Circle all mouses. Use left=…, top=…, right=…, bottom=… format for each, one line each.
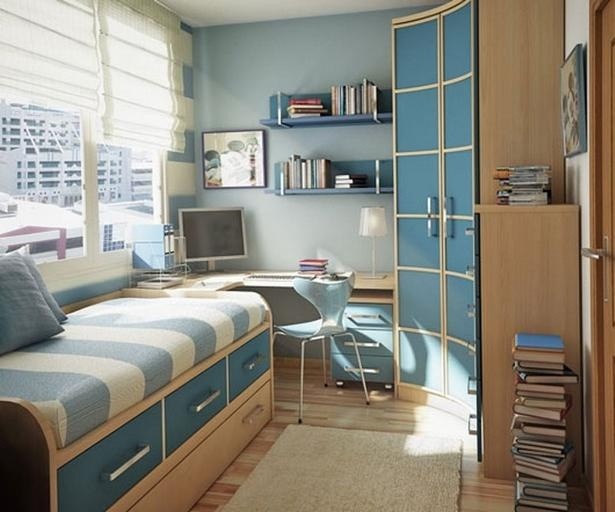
left=332, top=275, right=339, bottom=280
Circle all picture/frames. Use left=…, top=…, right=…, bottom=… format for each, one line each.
left=202, top=129, right=267, bottom=189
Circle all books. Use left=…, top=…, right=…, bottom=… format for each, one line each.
left=297, top=258, right=329, bottom=275
left=136, top=271, right=184, bottom=289
left=286, top=76, right=382, bottom=119
left=509, top=332, right=579, bottom=512
left=280, top=154, right=372, bottom=190
left=493, top=165, right=553, bottom=206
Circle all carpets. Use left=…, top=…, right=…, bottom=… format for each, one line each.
left=218, top=422, right=463, bottom=511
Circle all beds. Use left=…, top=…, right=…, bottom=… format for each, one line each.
left=0, top=287, right=273, bottom=512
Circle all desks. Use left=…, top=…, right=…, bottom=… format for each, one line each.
left=138, top=269, right=395, bottom=391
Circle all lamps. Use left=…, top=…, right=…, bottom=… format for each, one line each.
left=360, top=206, right=388, bottom=280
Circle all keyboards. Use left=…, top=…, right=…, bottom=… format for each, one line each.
left=243, top=274, right=316, bottom=282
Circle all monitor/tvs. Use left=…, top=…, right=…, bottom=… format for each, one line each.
left=178, top=206, right=249, bottom=274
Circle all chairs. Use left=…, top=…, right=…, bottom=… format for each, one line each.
left=270, top=273, right=370, bottom=424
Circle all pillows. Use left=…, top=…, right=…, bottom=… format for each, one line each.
left=0, top=242, right=69, bottom=355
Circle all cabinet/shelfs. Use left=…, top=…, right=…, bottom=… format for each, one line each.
left=391, top=2, right=564, bottom=415
left=466, top=201, right=582, bottom=480
